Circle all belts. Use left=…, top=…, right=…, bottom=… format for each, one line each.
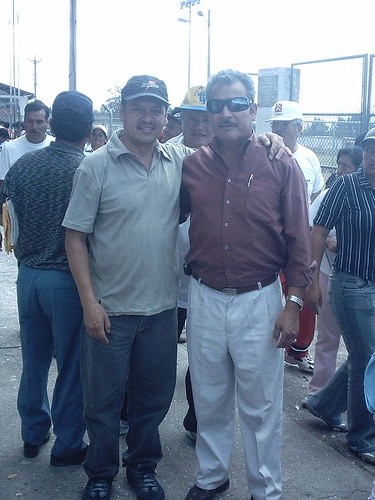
left=192, top=268, right=279, bottom=296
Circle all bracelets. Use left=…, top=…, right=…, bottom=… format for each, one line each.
left=286, top=293, right=305, bottom=308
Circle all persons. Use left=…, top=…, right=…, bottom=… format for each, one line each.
left=84, top=126, right=112, bottom=153
left=56, top=71, right=293, bottom=499
left=159, top=111, right=183, bottom=145
left=266, top=98, right=325, bottom=374
left=300, top=125, right=375, bottom=471
left=0, top=90, right=102, bottom=468
left=117, top=84, right=216, bottom=447
left=301, top=142, right=363, bottom=410
left=0, top=116, right=27, bottom=146
left=177, top=69, right=312, bottom=500
left=0, top=98, right=57, bottom=252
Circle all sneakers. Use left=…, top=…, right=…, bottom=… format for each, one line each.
left=126, top=466, right=164, bottom=500
left=83, top=477, right=112, bottom=500
left=284, top=350, right=314, bottom=372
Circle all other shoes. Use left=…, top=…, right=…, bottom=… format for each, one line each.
left=307, top=391, right=347, bottom=432
left=185, top=479, right=230, bottom=500
left=178, top=320, right=186, bottom=343
left=51, top=444, right=88, bottom=466
left=119, top=419, right=128, bottom=435
left=186, top=430, right=196, bottom=443
left=349, top=448, right=375, bottom=465
left=24, top=430, right=51, bottom=457
left=301, top=394, right=314, bottom=409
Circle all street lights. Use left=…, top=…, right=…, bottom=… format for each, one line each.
left=179, top=0, right=200, bottom=90
left=197, top=9, right=210, bottom=83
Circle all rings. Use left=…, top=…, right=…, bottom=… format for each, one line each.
left=291, top=338, right=298, bottom=344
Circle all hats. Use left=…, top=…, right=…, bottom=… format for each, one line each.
left=168, top=112, right=181, bottom=124
left=120, top=74, right=170, bottom=110
left=0, top=128, right=12, bottom=139
left=360, top=127, right=375, bottom=145
left=179, top=85, right=207, bottom=111
left=91, top=125, right=108, bottom=137
left=52, top=90, right=96, bottom=124
left=265, top=100, right=302, bottom=122
